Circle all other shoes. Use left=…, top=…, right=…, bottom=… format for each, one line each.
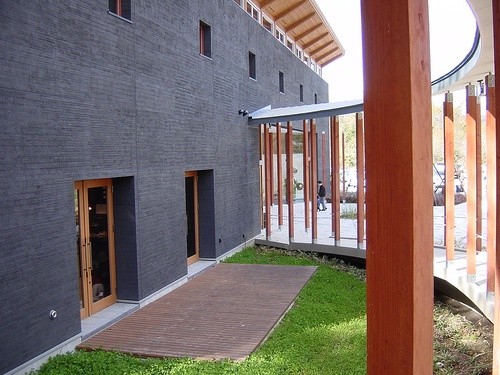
left=322, top=207, right=328, bottom=211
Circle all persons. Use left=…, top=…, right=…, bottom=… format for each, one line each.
left=317, top=194, right=321, bottom=211
left=317, top=181, right=328, bottom=211
